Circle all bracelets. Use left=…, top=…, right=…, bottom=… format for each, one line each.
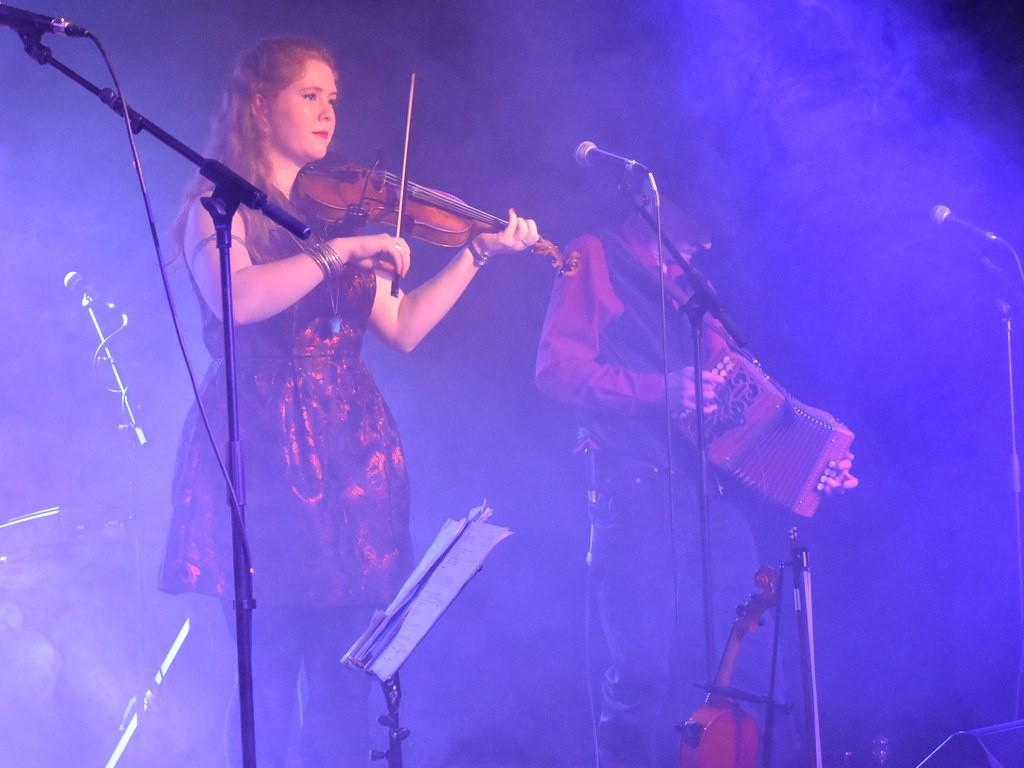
left=481, top=249, right=490, bottom=258
left=304, top=242, right=344, bottom=280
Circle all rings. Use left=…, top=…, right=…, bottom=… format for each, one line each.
left=521, top=240, right=528, bottom=247
left=394, top=243, right=402, bottom=250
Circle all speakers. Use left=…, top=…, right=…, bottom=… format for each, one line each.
left=916, top=719, right=1023, bottom=768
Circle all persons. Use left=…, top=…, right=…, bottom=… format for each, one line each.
left=158, top=35, right=538, bottom=767
left=534, top=144, right=858, bottom=767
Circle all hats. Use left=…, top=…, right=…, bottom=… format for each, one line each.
left=636, top=139, right=739, bottom=242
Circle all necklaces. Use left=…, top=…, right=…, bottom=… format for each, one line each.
left=279, top=225, right=342, bottom=336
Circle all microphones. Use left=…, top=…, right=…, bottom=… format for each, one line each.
left=64, top=271, right=118, bottom=312
left=573, top=140, right=650, bottom=176
left=0, top=3, right=89, bottom=37
left=930, top=205, right=1010, bottom=247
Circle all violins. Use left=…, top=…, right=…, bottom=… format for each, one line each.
left=674, top=565, right=780, bottom=768
left=295, top=152, right=583, bottom=279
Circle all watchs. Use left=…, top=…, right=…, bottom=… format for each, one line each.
left=467, top=241, right=488, bottom=268
